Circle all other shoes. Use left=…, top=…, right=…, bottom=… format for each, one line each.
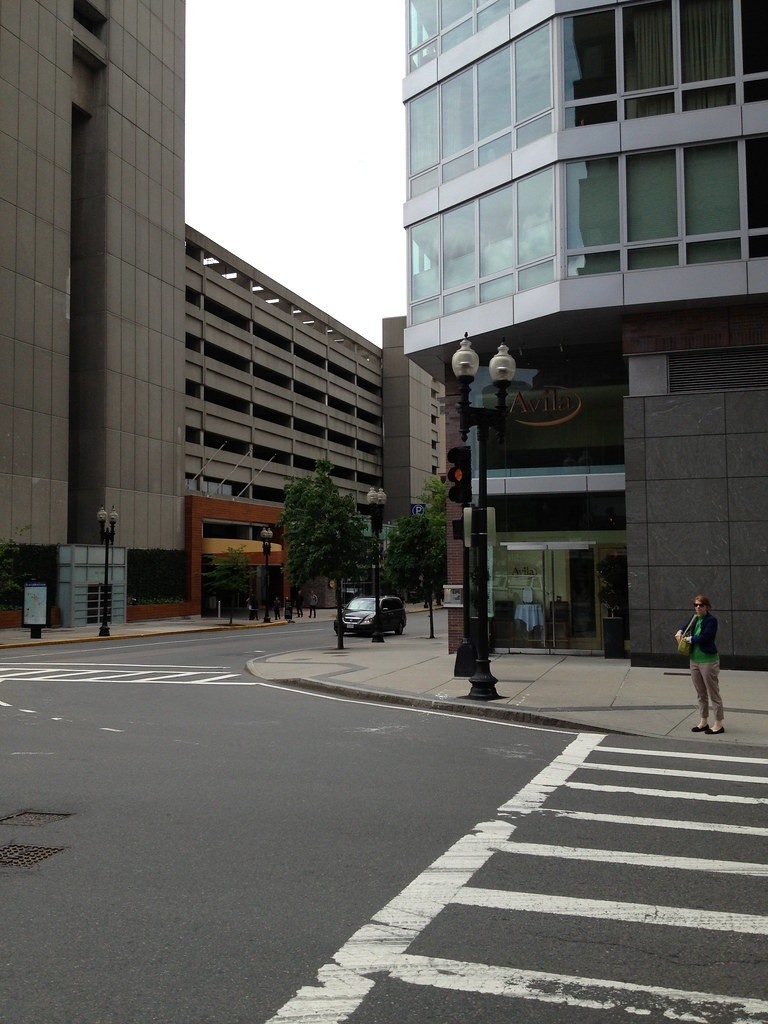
left=691, top=724, right=709, bottom=732
left=301, top=614, right=303, bottom=617
left=298, top=614, right=300, bottom=617
left=705, top=726, right=724, bottom=734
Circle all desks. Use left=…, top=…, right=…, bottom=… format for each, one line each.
left=514, top=604, right=544, bottom=648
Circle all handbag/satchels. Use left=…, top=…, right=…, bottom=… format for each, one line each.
left=678, top=614, right=696, bottom=656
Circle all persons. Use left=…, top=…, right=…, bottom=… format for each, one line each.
left=247, top=594, right=259, bottom=620
left=296, top=591, right=304, bottom=617
left=274, top=597, right=281, bottom=620
left=675, top=595, right=725, bottom=735
left=308, top=591, right=318, bottom=618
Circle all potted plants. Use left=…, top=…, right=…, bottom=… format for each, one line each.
left=596, top=555, right=628, bottom=659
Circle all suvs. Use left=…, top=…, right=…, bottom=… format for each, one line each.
left=333, top=595, right=407, bottom=636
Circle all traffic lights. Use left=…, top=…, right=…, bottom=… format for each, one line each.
left=446, top=446, right=473, bottom=504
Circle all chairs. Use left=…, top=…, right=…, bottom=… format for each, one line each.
left=491, top=599, right=515, bottom=648
left=546, top=600, right=570, bottom=646
left=527, top=604, right=548, bottom=648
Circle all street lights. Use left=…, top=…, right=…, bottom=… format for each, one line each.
left=260, top=528, right=273, bottom=623
left=366, top=487, right=386, bottom=643
left=450, top=329, right=518, bottom=699
left=99, top=505, right=119, bottom=636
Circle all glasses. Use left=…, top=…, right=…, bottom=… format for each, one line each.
left=693, top=603, right=706, bottom=607
